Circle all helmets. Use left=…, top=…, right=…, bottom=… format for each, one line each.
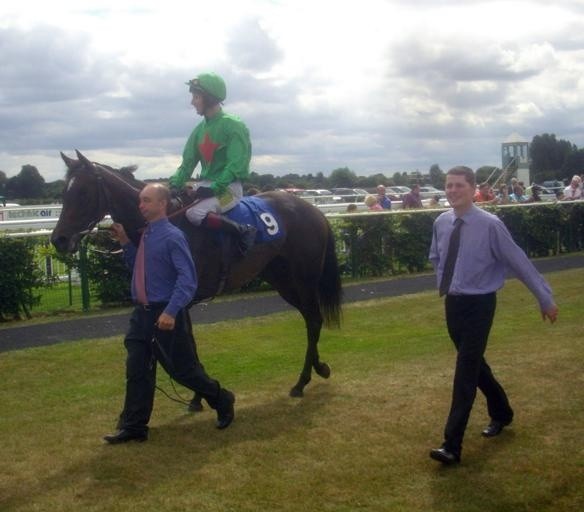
left=184, top=70, right=226, bottom=102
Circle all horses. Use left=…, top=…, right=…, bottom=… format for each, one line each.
left=49, top=147, right=344, bottom=414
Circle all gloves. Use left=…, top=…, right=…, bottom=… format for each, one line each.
left=197, top=186, right=213, bottom=199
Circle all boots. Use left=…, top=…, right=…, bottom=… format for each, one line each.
left=200, top=211, right=257, bottom=258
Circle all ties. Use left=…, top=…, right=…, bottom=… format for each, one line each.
left=439, top=218, right=464, bottom=297
left=135, top=227, right=149, bottom=305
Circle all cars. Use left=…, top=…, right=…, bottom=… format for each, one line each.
left=508, top=180, right=566, bottom=203
left=285, top=186, right=446, bottom=203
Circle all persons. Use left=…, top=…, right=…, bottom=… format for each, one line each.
left=162, top=73, right=257, bottom=259
left=102, top=182, right=235, bottom=444
left=344, top=183, right=442, bottom=212
left=474, top=175, right=584, bottom=206
left=428, top=166, right=558, bottom=464
left=242, top=182, right=278, bottom=197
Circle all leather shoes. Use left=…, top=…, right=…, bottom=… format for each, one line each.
left=482, top=417, right=513, bottom=437
left=104, top=427, right=149, bottom=444
left=216, top=388, right=235, bottom=430
left=430, top=445, right=462, bottom=466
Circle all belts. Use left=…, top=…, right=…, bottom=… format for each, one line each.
left=142, top=303, right=165, bottom=311
